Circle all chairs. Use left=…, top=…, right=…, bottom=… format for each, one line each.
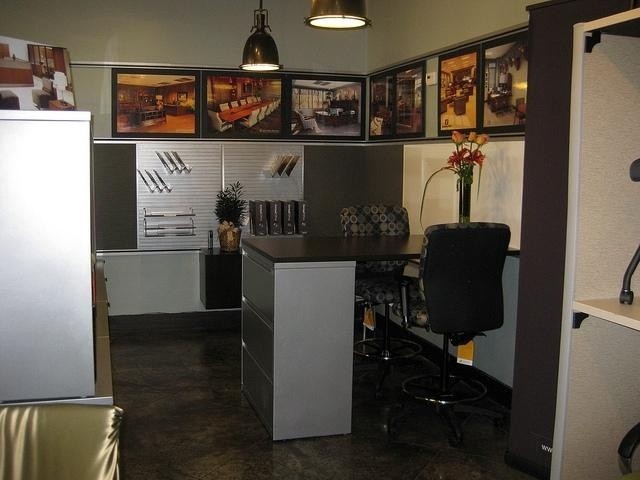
left=0, top=403, right=124, bottom=480
left=340, top=201, right=422, bottom=361
left=391, top=221, right=511, bottom=405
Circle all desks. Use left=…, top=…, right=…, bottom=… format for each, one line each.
left=32, top=89, right=74, bottom=110
left=0, top=90, right=20, bottom=110
left=241, top=234, right=522, bottom=441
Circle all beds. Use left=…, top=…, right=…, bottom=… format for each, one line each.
left=0, top=43, right=34, bottom=87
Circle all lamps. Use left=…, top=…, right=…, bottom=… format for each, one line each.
left=303, top=0, right=374, bottom=31
left=239, top=0, right=284, bottom=71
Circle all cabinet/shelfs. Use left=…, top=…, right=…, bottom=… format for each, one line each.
left=550, top=8, right=640, bottom=480
left=27, top=43, right=67, bottom=81
left=199, top=248, right=242, bottom=310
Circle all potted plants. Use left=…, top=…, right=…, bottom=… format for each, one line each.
left=213, top=179, right=249, bottom=253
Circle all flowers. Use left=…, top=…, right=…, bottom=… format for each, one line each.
left=417, top=131, right=490, bottom=227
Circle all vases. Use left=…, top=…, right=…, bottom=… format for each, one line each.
left=459, top=174, right=472, bottom=224
